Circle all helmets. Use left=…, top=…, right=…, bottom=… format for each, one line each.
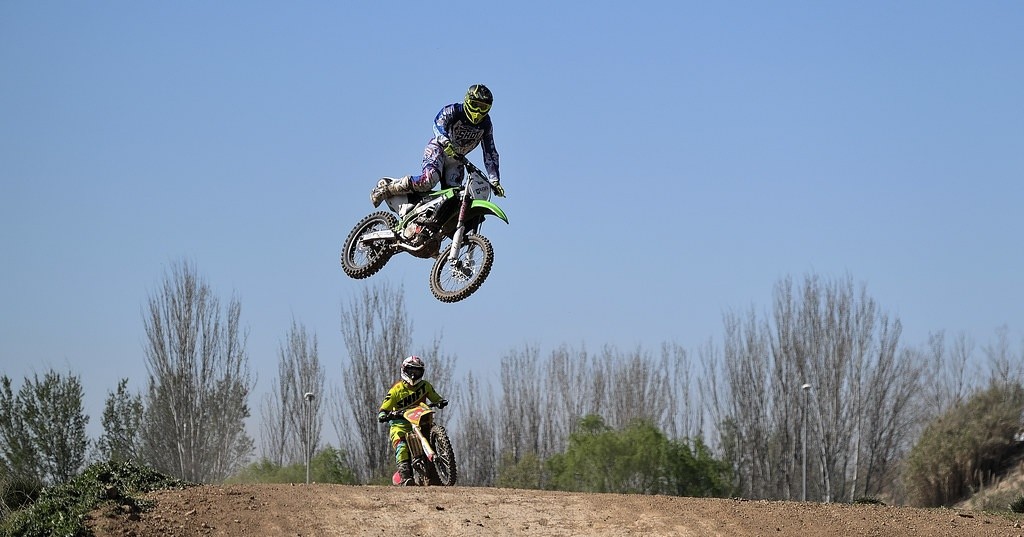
left=400, top=355, right=425, bottom=386
left=463, top=84, right=493, bottom=124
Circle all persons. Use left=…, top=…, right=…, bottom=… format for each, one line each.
left=379, top=356, right=448, bottom=486
left=371, top=85, right=504, bottom=207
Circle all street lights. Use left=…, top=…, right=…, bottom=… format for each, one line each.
left=801, top=383, right=814, bottom=502
left=303, top=391, right=316, bottom=484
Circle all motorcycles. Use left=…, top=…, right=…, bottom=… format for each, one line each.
left=340, top=146, right=509, bottom=304
left=379, top=401, right=458, bottom=487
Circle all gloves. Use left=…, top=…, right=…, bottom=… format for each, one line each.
left=437, top=400, right=448, bottom=409
left=378, top=411, right=387, bottom=423
left=444, top=143, right=455, bottom=158
left=492, top=181, right=504, bottom=197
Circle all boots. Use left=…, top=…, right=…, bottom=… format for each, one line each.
left=370, top=176, right=409, bottom=208
left=397, top=460, right=415, bottom=487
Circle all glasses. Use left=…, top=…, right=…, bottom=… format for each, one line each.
left=405, top=367, right=423, bottom=377
left=469, top=100, right=491, bottom=112
